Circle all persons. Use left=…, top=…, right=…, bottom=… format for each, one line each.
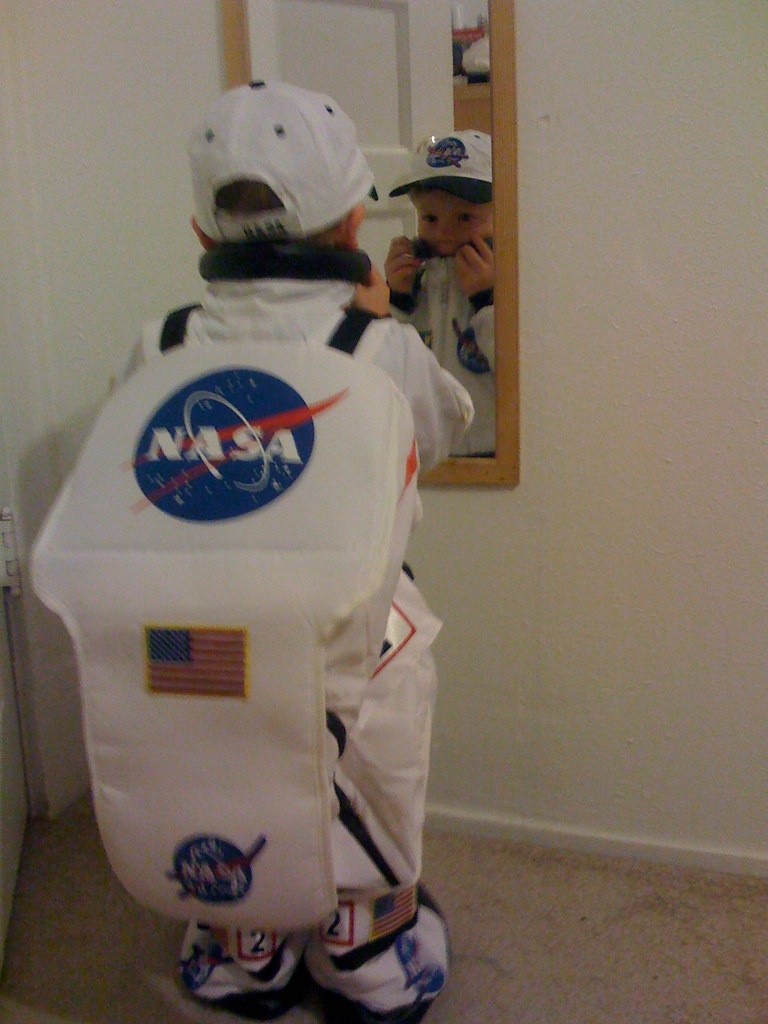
left=383, top=129, right=496, bottom=458
left=28, top=80, right=475, bottom=1024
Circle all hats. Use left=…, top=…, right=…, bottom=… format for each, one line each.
left=388, top=128, right=492, bottom=204
left=187, top=79, right=378, bottom=243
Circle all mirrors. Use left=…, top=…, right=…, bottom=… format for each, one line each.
left=221, top=0, right=521, bottom=493
